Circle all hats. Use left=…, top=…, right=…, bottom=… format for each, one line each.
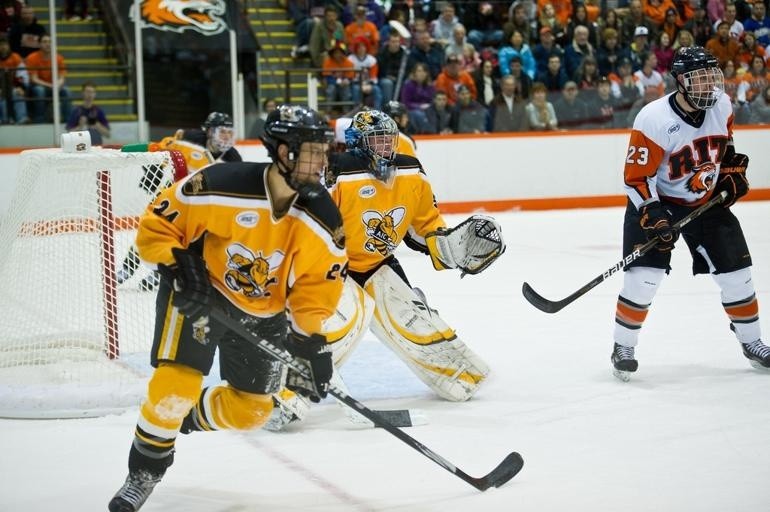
left=634, top=26, right=648, bottom=36
left=446, top=56, right=459, bottom=64
left=564, top=81, right=576, bottom=89
left=541, top=27, right=551, bottom=34
left=666, top=6, right=676, bottom=16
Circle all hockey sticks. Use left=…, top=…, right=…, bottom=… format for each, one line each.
left=330, top=366, right=431, bottom=428
left=390, top=20, right=411, bottom=101
left=158, top=262, right=524, bottom=491
left=522, top=189, right=729, bottom=314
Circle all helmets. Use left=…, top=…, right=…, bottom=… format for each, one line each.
left=671, top=46, right=723, bottom=111
left=260, top=104, right=336, bottom=196
left=345, top=109, right=399, bottom=190
left=204, top=112, right=235, bottom=152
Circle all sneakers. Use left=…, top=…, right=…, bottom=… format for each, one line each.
left=141, top=269, right=162, bottom=291
left=114, top=268, right=134, bottom=282
left=742, top=340, right=770, bottom=366
left=611, top=343, right=637, bottom=374
left=109, top=472, right=164, bottom=512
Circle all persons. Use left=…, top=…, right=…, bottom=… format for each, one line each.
left=0, top=0, right=68, bottom=125
left=261, top=109, right=506, bottom=431
left=260, top=99, right=276, bottom=121
left=298, top=1, right=769, bottom=134
left=65, top=82, right=112, bottom=146
left=115, top=111, right=244, bottom=290
left=327, top=101, right=437, bottom=256
left=611, top=46, right=770, bottom=381
left=108, top=106, right=350, bottom=512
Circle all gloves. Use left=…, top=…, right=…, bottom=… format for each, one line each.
left=426, top=216, right=508, bottom=274
left=720, top=154, right=750, bottom=208
left=160, top=245, right=215, bottom=321
left=641, top=201, right=679, bottom=252
left=282, top=331, right=332, bottom=404
